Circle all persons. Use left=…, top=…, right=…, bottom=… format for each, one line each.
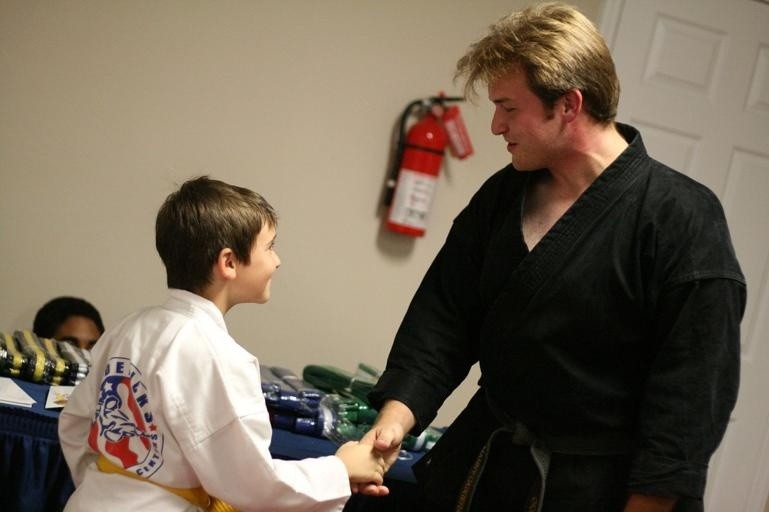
left=33, top=296, right=104, bottom=351
left=57, top=174, right=386, bottom=512
left=350, top=0, right=747, bottom=512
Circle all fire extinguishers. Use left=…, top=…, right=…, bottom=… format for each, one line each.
left=381, top=94, right=474, bottom=238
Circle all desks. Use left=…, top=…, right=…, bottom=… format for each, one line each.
left=0, top=377, right=449, bottom=511
